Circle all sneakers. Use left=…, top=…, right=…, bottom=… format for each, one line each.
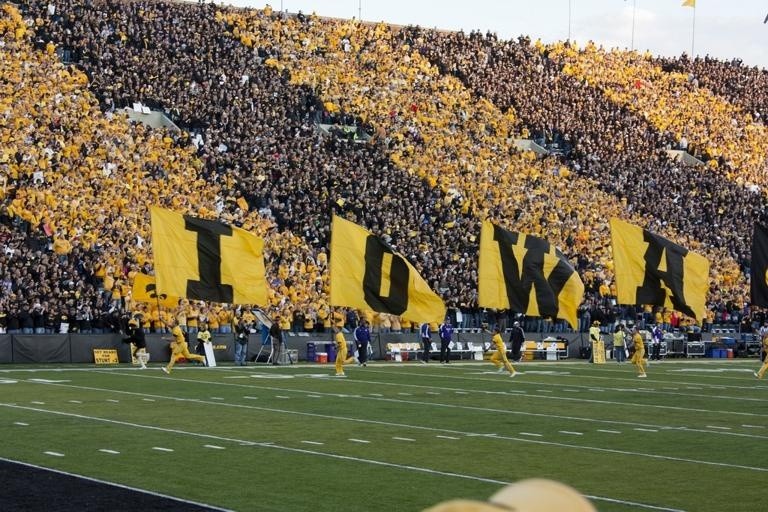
left=753, top=372, right=759, bottom=379
left=203, top=357, right=206, bottom=366
left=498, top=365, right=517, bottom=378
left=160, top=366, right=169, bottom=375
left=336, top=357, right=360, bottom=376
left=637, top=359, right=649, bottom=377
left=139, top=353, right=150, bottom=370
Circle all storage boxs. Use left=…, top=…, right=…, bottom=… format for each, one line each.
left=705, top=333, right=753, bottom=358
left=315, top=352, right=328, bottom=364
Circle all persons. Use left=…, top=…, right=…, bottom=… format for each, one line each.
left=588, top=321, right=603, bottom=362
left=268, top=315, right=283, bottom=366
left=753, top=325, right=768, bottom=380
left=509, top=321, right=526, bottom=362
left=353, top=319, right=371, bottom=367
left=121, top=318, right=150, bottom=369
left=159, top=315, right=207, bottom=375
left=194, top=323, right=212, bottom=365
left=234, top=316, right=249, bottom=367
left=0, top=0, right=767, bottom=335
left=650, top=322, right=664, bottom=361
left=420, top=322, right=432, bottom=363
left=622, top=325, right=649, bottom=379
left=328, top=318, right=361, bottom=377
left=483, top=327, right=517, bottom=379
left=759, top=320, right=768, bottom=348
left=439, top=315, right=451, bottom=364
left=611, top=325, right=627, bottom=365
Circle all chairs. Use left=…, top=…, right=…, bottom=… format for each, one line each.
left=386, top=339, right=568, bottom=361
